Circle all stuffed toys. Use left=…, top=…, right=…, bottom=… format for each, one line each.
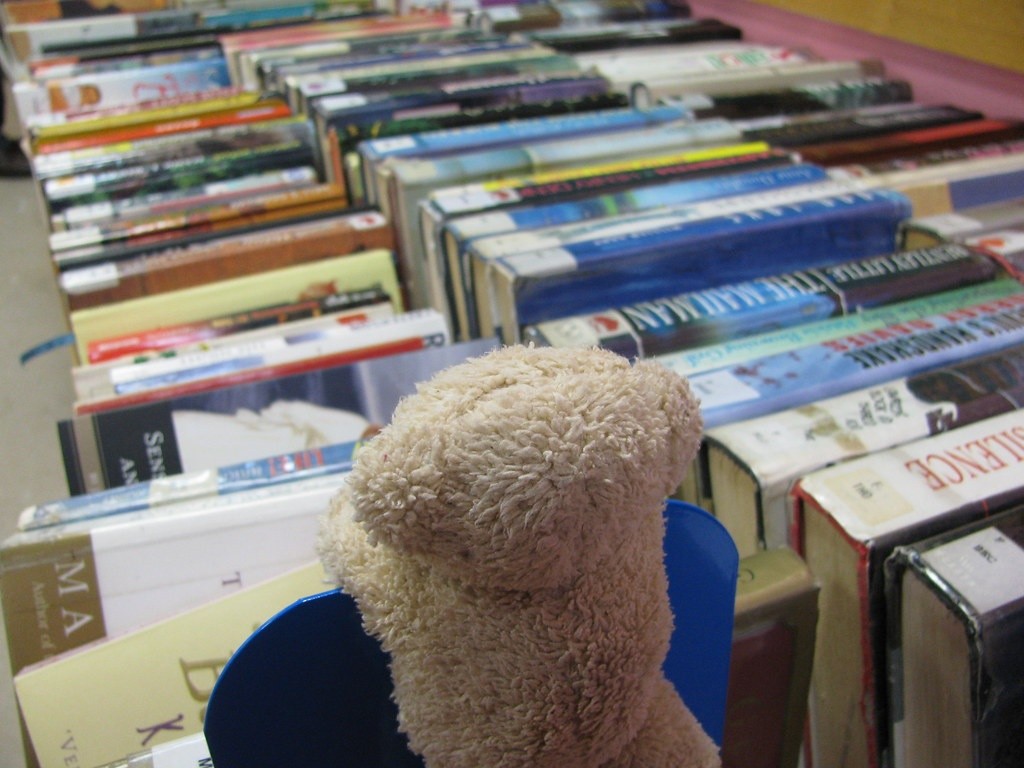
left=315, top=346, right=722, bottom=768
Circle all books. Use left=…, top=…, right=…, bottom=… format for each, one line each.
left=0, top=0, right=1024, bottom=768
